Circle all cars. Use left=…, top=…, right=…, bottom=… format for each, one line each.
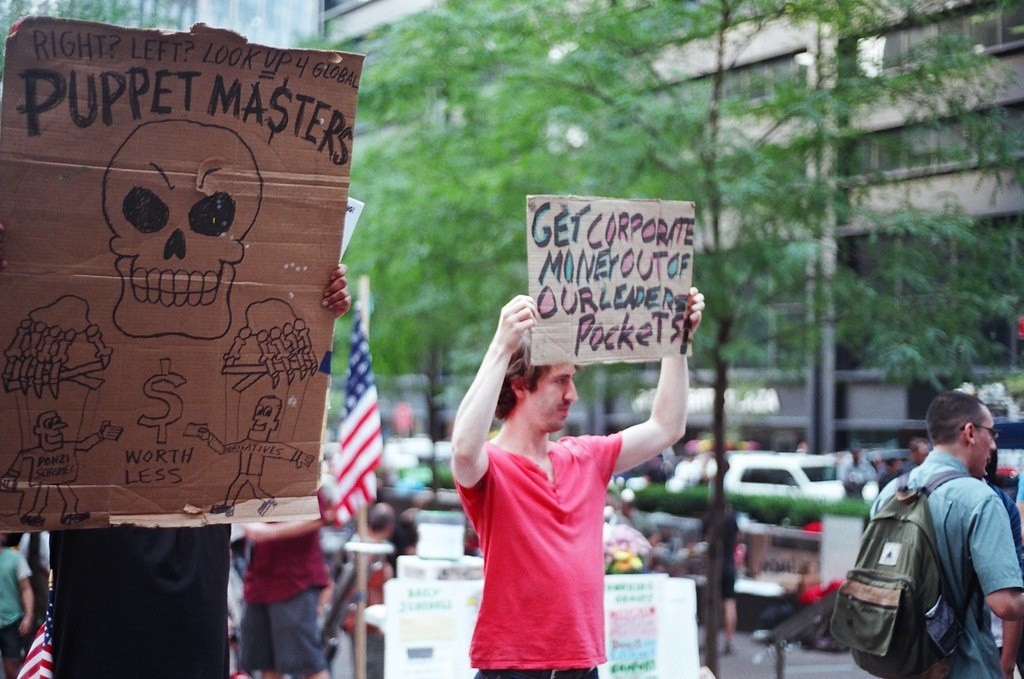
left=724, top=450, right=848, bottom=498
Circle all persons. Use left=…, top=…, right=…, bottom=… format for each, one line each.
left=834, top=387, right=1023, bottom=679
left=235, top=486, right=334, bottom=679
left=0, top=533, right=38, bottom=678
left=602, top=420, right=931, bottom=654
left=335, top=501, right=398, bottom=637
left=436, top=282, right=709, bottom=677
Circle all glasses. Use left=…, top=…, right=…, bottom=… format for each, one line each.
left=961, top=422, right=1001, bottom=440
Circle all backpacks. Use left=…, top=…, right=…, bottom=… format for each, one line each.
left=832, top=471, right=973, bottom=679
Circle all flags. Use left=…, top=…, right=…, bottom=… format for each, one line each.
left=331, top=297, right=386, bottom=532
left=16, top=571, right=54, bottom=679
